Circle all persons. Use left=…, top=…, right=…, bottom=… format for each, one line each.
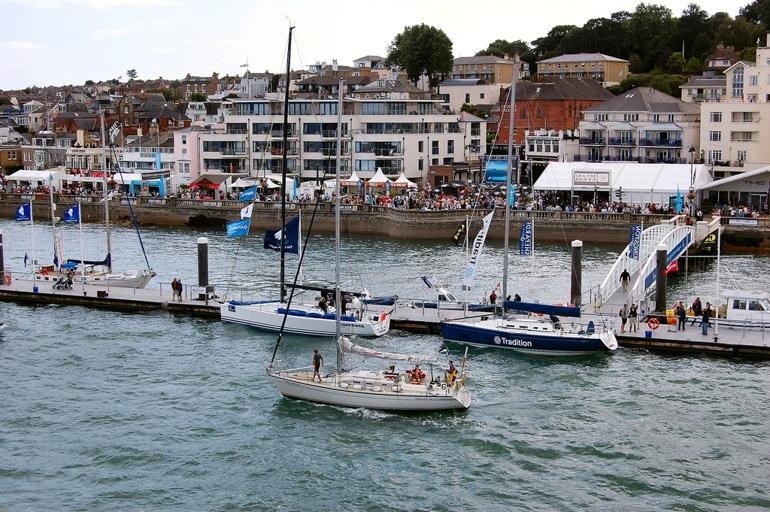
left=172, top=278, right=179, bottom=301
left=514, top=294, right=521, bottom=301
left=627, top=304, right=638, bottom=332
left=318, top=293, right=348, bottom=316
left=446, top=360, right=458, bottom=386
left=675, top=297, right=712, bottom=336
left=121, top=191, right=160, bottom=200
left=490, top=290, right=496, bottom=304
left=16, top=184, right=85, bottom=198
left=621, top=304, right=629, bottom=333
left=410, top=365, right=423, bottom=384
left=311, top=348, right=323, bottom=382
left=299, top=189, right=758, bottom=225
left=176, top=188, right=236, bottom=203
left=178, top=280, right=182, bottom=301
left=619, top=269, right=630, bottom=292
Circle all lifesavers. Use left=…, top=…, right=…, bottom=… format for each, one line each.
left=648, top=318, right=660, bottom=330
left=4, top=276, right=12, bottom=283
left=382, top=312, right=386, bottom=320
left=42, top=267, right=49, bottom=275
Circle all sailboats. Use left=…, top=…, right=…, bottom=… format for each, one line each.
left=1, top=106, right=156, bottom=290
left=265, top=76, right=471, bottom=417
left=444, top=57, right=618, bottom=361
left=220, top=25, right=392, bottom=342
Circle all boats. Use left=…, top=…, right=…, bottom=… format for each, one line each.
left=665, top=307, right=695, bottom=324
left=724, top=289, right=770, bottom=327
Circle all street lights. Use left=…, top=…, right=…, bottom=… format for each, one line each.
left=688, top=145, right=697, bottom=222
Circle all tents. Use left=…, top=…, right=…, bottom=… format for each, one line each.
left=533, top=162, right=714, bottom=211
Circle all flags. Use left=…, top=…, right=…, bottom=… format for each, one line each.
left=16, top=204, right=30, bottom=221
left=240, top=202, right=253, bottom=219
left=227, top=219, right=249, bottom=238
left=239, top=187, right=256, bottom=203
left=263, top=216, right=298, bottom=254
left=63, top=204, right=79, bottom=224
